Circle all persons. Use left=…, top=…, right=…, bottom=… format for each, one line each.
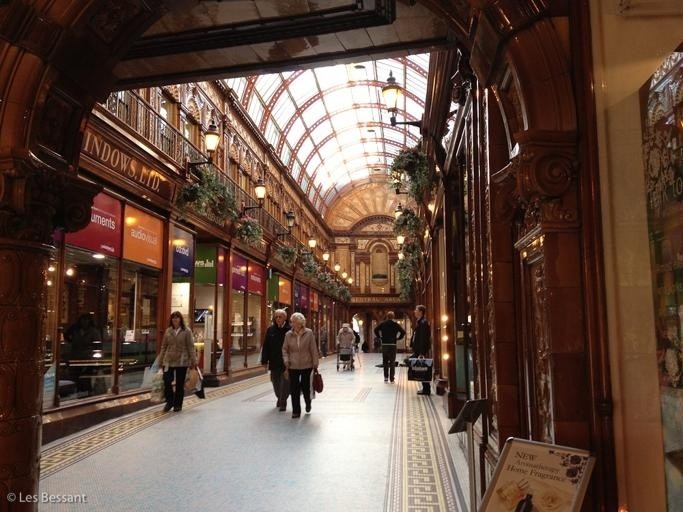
left=260, top=309, right=291, bottom=411
left=362, top=340, right=370, bottom=352
left=320, top=326, right=328, bottom=359
left=373, top=311, right=406, bottom=382
left=69, top=312, right=104, bottom=397
left=336, top=323, right=356, bottom=370
left=352, top=329, right=361, bottom=368
left=282, top=312, right=319, bottom=418
left=159, top=309, right=199, bottom=411
left=409, top=305, right=432, bottom=395
left=373, top=336, right=381, bottom=353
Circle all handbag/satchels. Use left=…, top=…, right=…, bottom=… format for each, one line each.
left=184, top=365, right=205, bottom=399
left=313, top=373, right=323, bottom=393
left=408, top=358, right=433, bottom=381
left=150, top=371, right=165, bottom=402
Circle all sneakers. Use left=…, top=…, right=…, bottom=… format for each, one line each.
left=163, top=401, right=181, bottom=412
left=277, top=398, right=311, bottom=418
left=384, top=378, right=394, bottom=382
left=417, top=391, right=430, bottom=395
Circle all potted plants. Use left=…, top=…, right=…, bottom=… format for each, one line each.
left=177, top=184, right=207, bottom=217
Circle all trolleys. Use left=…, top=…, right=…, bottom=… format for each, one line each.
left=336, top=342, right=356, bottom=372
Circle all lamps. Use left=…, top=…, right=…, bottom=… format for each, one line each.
left=276, top=208, right=296, bottom=240
left=185, top=120, right=220, bottom=179
left=382, top=70, right=423, bottom=134
left=394, top=177, right=406, bottom=263
left=301, top=235, right=353, bottom=285
left=242, top=176, right=266, bottom=216
